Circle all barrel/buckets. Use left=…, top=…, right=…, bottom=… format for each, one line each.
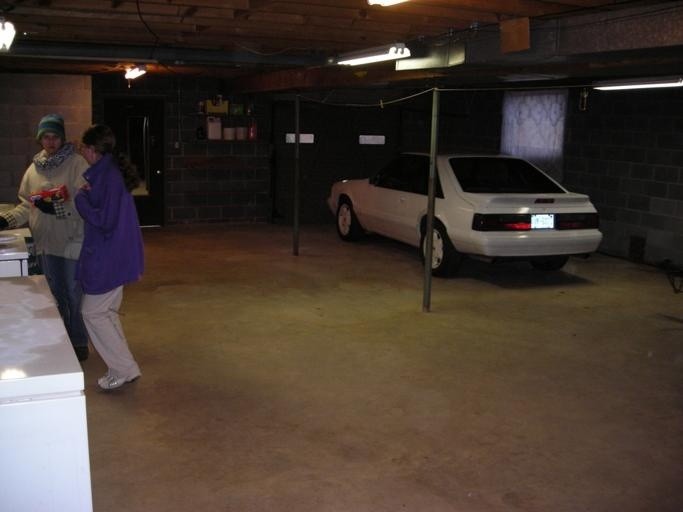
left=236, top=126, right=246, bottom=140
left=245, top=112, right=258, bottom=139
left=224, top=127, right=235, bottom=140
left=206, top=116, right=222, bottom=140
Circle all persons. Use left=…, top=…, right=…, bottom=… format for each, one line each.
left=0, top=113, right=92, bottom=362
left=73, top=124, right=144, bottom=391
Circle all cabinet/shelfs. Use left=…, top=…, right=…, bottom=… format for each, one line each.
left=186, top=112, right=260, bottom=145
left=0, top=227, right=32, bottom=278
left=0, top=271, right=96, bottom=512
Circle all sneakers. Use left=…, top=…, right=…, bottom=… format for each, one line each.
left=97, top=369, right=143, bottom=390
left=74, top=346, right=90, bottom=361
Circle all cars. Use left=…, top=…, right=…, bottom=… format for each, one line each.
left=326, top=148, right=607, bottom=274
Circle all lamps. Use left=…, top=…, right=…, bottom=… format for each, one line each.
left=334, top=42, right=412, bottom=70
left=590, top=76, right=682, bottom=93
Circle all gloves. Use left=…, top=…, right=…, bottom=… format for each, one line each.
left=34, top=199, right=56, bottom=214
left=0, top=216, right=9, bottom=228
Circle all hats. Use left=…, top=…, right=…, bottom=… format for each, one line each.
left=34, top=113, right=65, bottom=145
